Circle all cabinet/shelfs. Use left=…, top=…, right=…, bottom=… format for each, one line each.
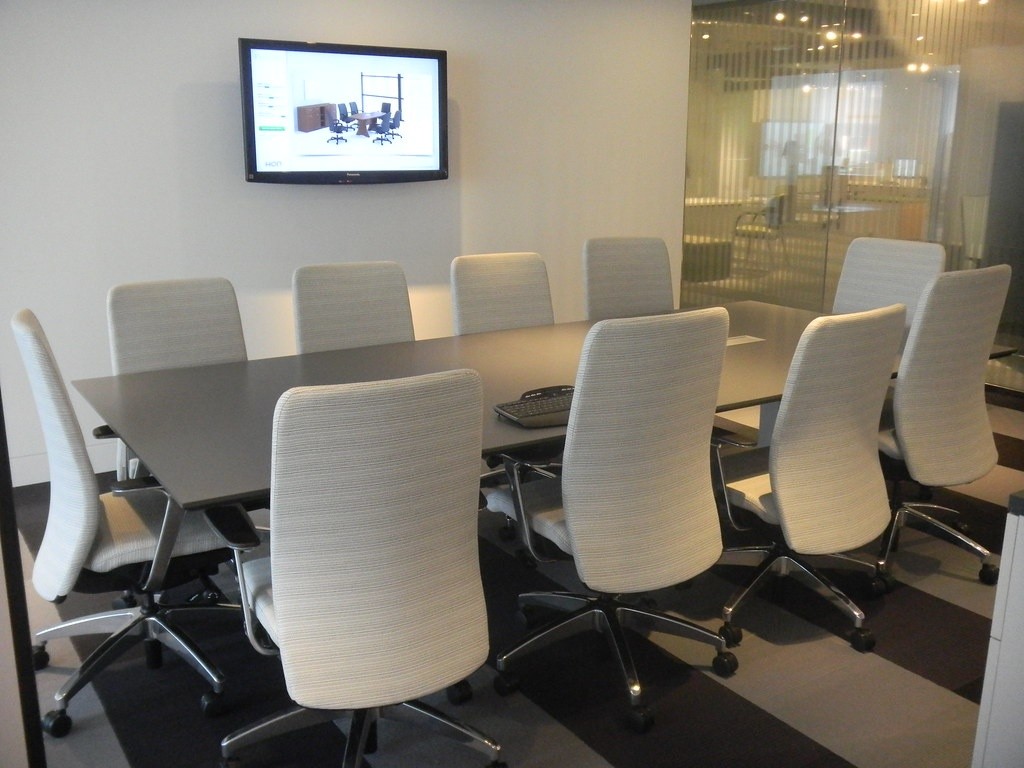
left=297, top=103, right=336, bottom=132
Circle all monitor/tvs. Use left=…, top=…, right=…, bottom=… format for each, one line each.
left=237, top=38, right=448, bottom=184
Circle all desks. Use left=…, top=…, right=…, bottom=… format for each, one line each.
left=781, top=193, right=928, bottom=286
left=71, top=300, right=1018, bottom=509
left=684, top=196, right=766, bottom=239
left=348, top=112, right=386, bottom=137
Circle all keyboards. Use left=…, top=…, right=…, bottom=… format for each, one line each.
left=494, top=385, right=576, bottom=428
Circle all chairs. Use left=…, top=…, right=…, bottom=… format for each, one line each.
left=11, top=237, right=1012, bottom=768
left=325, top=102, right=402, bottom=145
left=732, top=195, right=788, bottom=271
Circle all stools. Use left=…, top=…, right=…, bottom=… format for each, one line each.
left=683, top=237, right=732, bottom=282
